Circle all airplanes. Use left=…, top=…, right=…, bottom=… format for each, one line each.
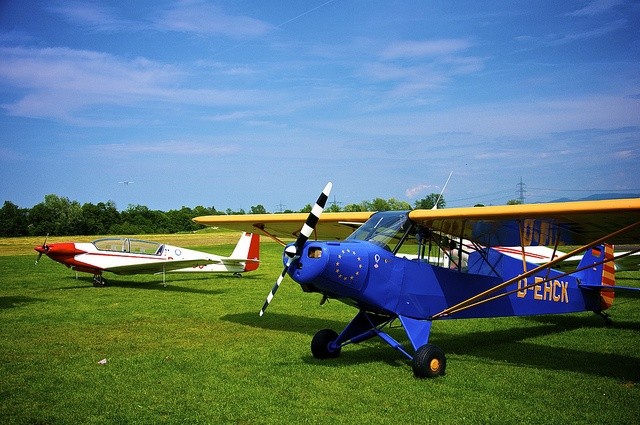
left=394, top=236, right=640, bottom=269
left=190, top=172, right=640, bottom=380
left=33, top=231, right=260, bottom=288
